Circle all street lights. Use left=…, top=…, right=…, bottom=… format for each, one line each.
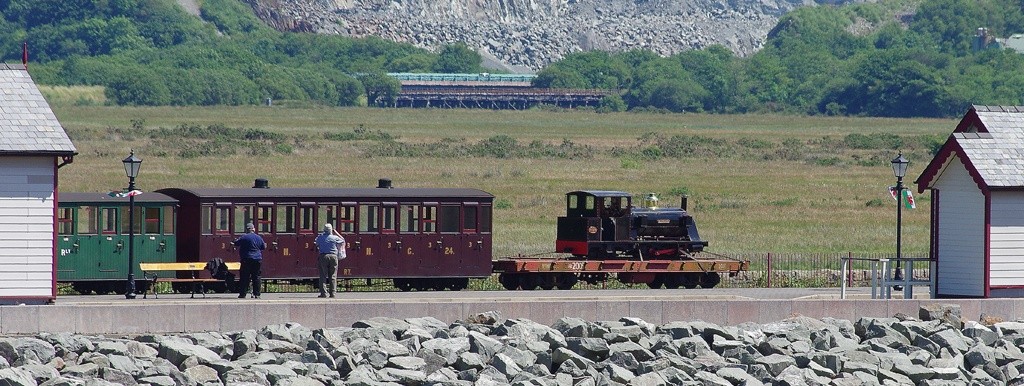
left=121, top=149, right=144, bottom=299
left=891, top=150, right=911, bottom=291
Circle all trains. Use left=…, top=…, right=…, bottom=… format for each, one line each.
left=58, top=175, right=751, bottom=289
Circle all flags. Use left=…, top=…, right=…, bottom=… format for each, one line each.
left=108, top=190, right=144, bottom=198
left=889, top=186, right=903, bottom=201
left=903, top=189, right=916, bottom=209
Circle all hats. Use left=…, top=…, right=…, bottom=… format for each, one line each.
left=245, top=223, right=254, bottom=229
left=324, top=224, right=333, bottom=231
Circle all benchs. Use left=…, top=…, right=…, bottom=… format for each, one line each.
left=140, top=262, right=242, bottom=298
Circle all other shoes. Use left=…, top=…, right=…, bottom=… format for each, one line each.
left=238, top=295, right=246, bottom=299
left=329, top=294, right=335, bottom=298
left=318, top=292, right=327, bottom=298
left=254, top=296, right=261, bottom=299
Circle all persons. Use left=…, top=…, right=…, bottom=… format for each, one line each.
left=234, top=223, right=267, bottom=298
left=316, top=223, right=344, bottom=298
